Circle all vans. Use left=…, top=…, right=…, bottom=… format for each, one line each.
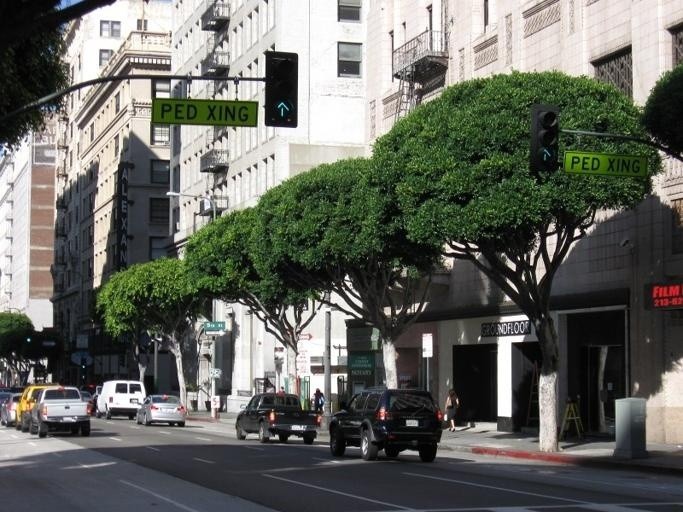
left=97, top=379, right=147, bottom=420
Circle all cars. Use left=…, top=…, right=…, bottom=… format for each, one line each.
left=136, top=394, right=185, bottom=427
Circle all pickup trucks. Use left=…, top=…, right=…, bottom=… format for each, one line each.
left=0, top=383, right=103, bottom=438
left=236, top=392, right=322, bottom=444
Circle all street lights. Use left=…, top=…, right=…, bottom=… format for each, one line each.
left=166, top=191, right=218, bottom=420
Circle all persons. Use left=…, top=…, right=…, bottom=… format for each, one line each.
left=313, top=388, right=323, bottom=412
left=279, top=386, right=286, bottom=393
left=444, top=390, right=461, bottom=432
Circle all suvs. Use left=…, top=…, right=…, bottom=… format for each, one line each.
left=329, top=389, right=444, bottom=462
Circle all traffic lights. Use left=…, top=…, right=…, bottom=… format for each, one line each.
left=529, top=103, right=559, bottom=170
left=264, top=51, right=298, bottom=128
